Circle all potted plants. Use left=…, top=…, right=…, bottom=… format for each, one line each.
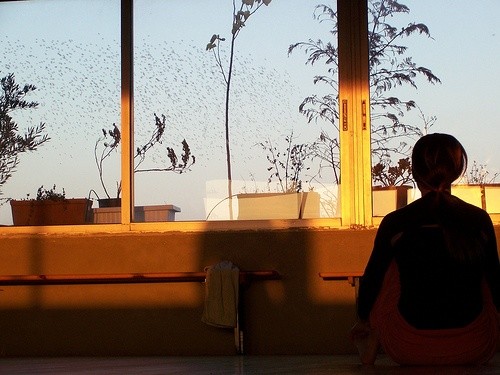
left=451, top=162, right=500, bottom=214
left=94, top=113, right=196, bottom=224
left=238, top=129, right=321, bottom=219
left=10, top=183, right=94, bottom=226
left=371, top=157, right=417, bottom=217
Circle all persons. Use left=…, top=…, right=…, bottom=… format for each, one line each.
left=349, top=133, right=500, bottom=365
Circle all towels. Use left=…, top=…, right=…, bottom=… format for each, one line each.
left=200, top=261, right=240, bottom=328
